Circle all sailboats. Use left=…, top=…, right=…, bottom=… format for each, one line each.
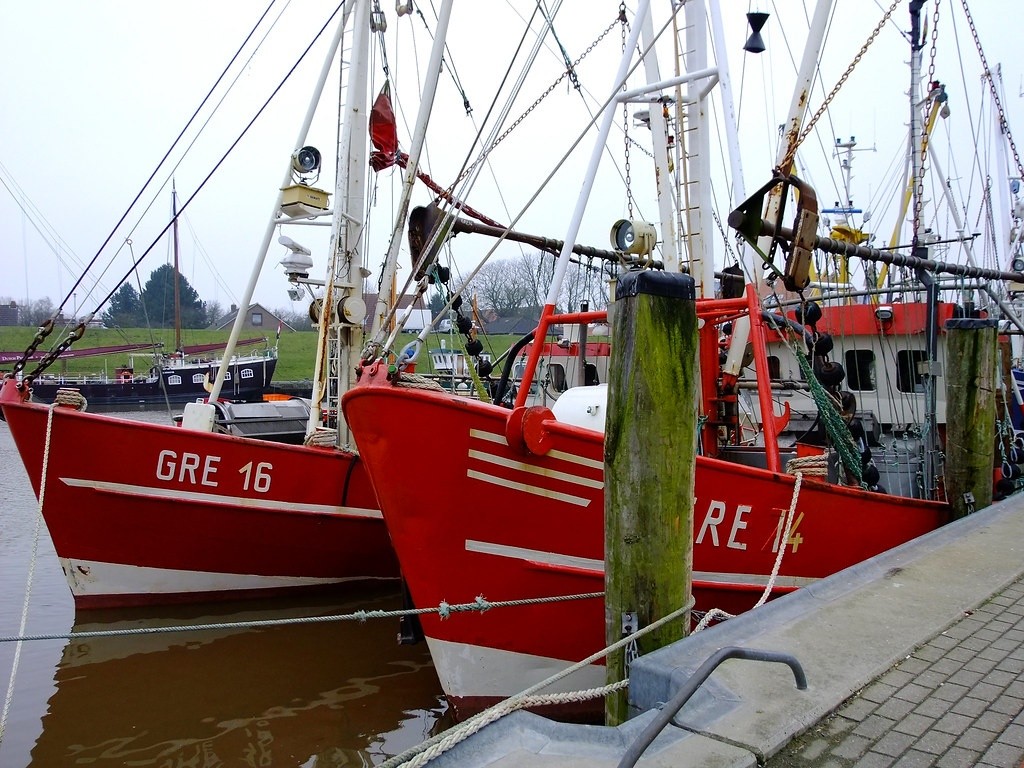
left=1, top=0, right=1024, bottom=717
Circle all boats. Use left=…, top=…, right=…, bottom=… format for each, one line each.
left=2, top=319, right=280, bottom=405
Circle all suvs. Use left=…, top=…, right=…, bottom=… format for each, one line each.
left=440, top=319, right=478, bottom=333
